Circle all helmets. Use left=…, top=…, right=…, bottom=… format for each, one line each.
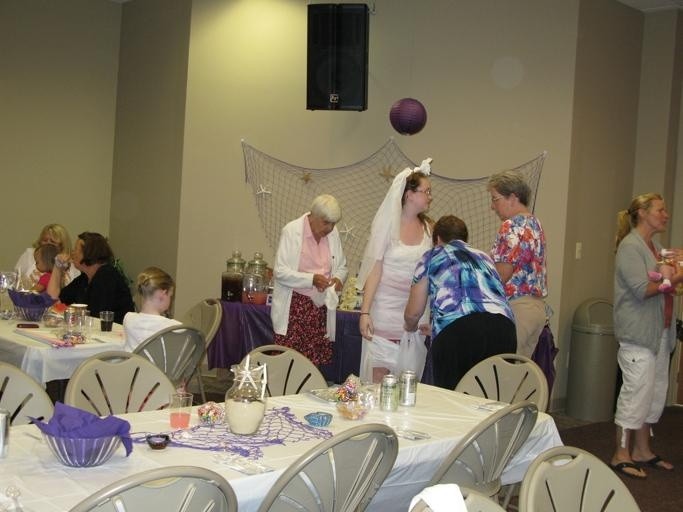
left=491, top=196, right=505, bottom=204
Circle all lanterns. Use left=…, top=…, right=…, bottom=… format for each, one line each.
left=390, top=97, right=427, bottom=137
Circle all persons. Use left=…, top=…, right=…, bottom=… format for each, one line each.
left=270, top=194, right=349, bottom=373
left=122, top=266, right=188, bottom=395
left=609, top=192, right=676, bottom=480
left=486, top=169, right=548, bottom=368
left=26, top=243, right=65, bottom=298
left=404, top=214, right=517, bottom=397
left=11, top=223, right=81, bottom=290
left=353, top=158, right=436, bottom=387
left=647, top=248, right=683, bottom=293
left=45, top=231, right=133, bottom=327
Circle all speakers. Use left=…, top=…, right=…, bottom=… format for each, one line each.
left=306, top=4, right=369, bottom=111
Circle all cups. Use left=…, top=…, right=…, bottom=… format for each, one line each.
left=170, top=392, right=192, bottom=428
left=76, top=315, right=98, bottom=338
left=0, top=273, right=17, bottom=318
left=98, top=310, right=113, bottom=331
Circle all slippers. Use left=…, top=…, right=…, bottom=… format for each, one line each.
left=608, top=459, right=648, bottom=480
left=631, top=454, right=674, bottom=470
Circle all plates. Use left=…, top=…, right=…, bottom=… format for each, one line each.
left=307, top=388, right=339, bottom=404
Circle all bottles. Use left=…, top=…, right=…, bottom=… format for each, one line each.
left=223, top=365, right=264, bottom=433
left=221, top=251, right=267, bottom=301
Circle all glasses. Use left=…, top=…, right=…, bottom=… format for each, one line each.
left=413, top=189, right=431, bottom=195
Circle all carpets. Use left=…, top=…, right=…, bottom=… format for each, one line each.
left=488, top=404, right=682, bottom=512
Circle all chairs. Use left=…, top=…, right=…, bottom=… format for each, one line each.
left=2, top=300, right=549, bottom=510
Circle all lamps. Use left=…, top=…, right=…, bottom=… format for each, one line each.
left=387, top=1, right=427, bottom=138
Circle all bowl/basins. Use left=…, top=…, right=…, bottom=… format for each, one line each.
left=40, top=432, right=122, bottom=466
left=335, top=398, right=374, bottom=419
left=42, top=315, right=58, bottom=327
left=12, top=303, right=49, bottom=321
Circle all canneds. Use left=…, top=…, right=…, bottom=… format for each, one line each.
left=400, top=370, right=417, bottom=407
left=380, top=375, right=399, bottom=412
left=0, top=410, right=10, bottom=459
left=64, top=307, right=76, bottom=325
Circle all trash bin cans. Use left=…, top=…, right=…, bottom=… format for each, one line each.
left=565, top=297, right=619, bottom=422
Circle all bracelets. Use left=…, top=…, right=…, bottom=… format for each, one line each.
left=360, top=313, right=369, bottom=316
